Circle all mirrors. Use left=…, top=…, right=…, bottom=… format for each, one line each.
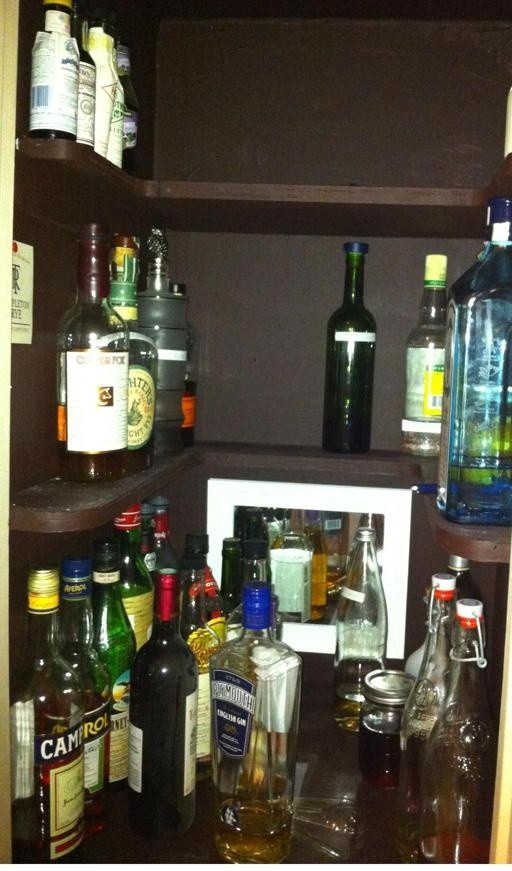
left=205, top=477, right=414, bottom=661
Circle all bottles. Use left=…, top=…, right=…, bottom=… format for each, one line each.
left=27, top=0, right=139, bottom=175
left=401, top=197, right=512, bottom=529
left=56, top=222, right=197, bottom=484
left=234, top=505, right=384, bottom=625
left=322, top=242, right=377, bottom=454
left=9, top=498, right=497, bottom=864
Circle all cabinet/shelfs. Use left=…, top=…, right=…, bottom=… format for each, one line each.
left=2, top=2, right=511, bottom=864
